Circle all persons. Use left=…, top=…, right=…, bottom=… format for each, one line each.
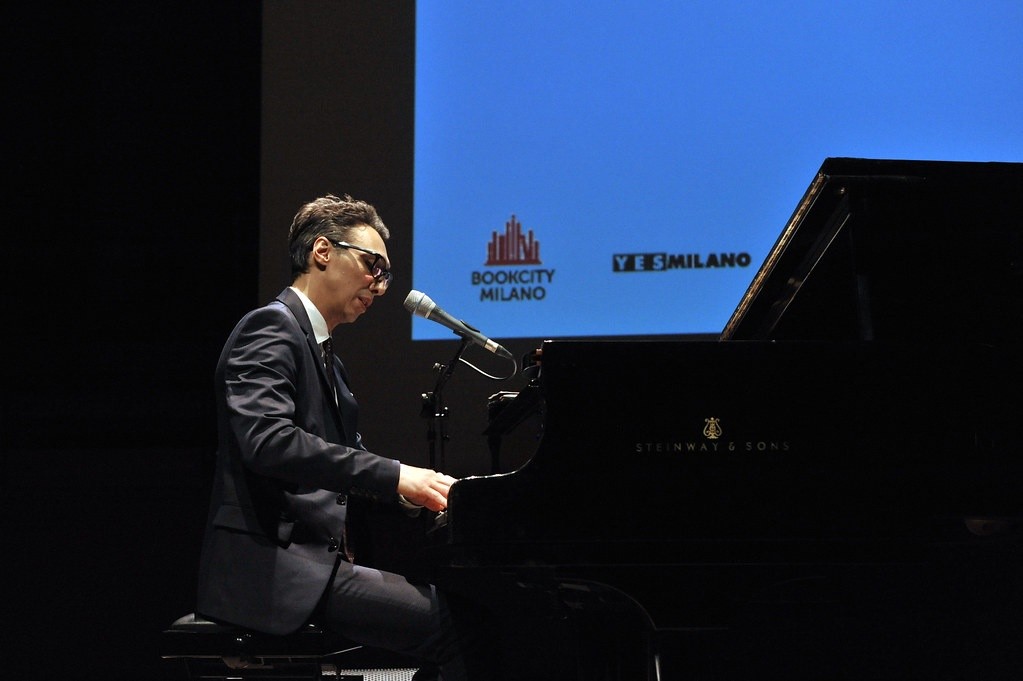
left=195, top=195, right=493, bottom=680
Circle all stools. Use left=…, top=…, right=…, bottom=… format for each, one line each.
left=159, top=612, right=363, bottom=681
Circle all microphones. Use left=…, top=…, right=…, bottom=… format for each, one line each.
left=403, top=290, right=514, bottom=359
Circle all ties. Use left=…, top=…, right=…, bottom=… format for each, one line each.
left=323, top=337, right=335, bottom=398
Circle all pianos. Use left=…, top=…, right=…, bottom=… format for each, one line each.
left=376, top=154, right=1023, bottom=678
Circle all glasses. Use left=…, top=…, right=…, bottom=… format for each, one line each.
left=331, top=240, right=393, bottom=289
